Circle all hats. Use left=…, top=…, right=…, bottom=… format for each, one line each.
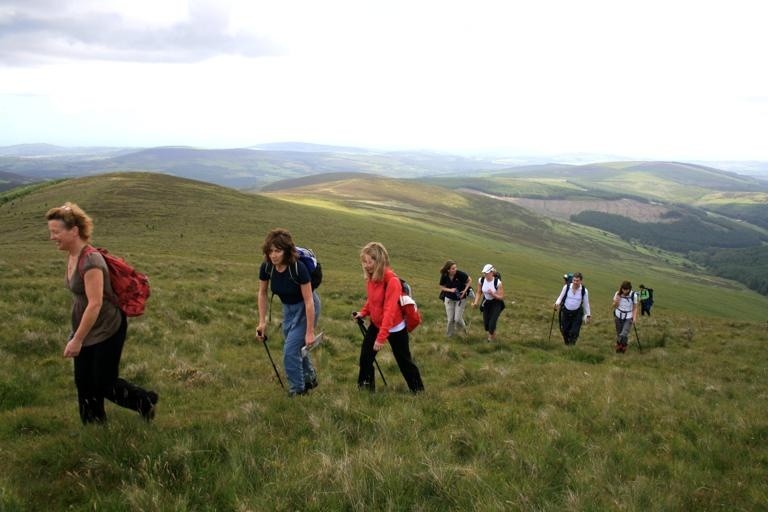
left=481, top=263, right=496, bottom=274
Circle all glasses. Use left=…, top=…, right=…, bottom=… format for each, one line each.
left=58, top=204, right=74, bottom=215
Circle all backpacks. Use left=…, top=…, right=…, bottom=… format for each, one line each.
left=96, top=247, right=150, bottom=318
left=562, top=272, right=574, bottom=283
left=400, top=278, right=424, bottom=334
left=294, top=246, right=322, bottom=291
left=495, top=272, right=502, bottom=281
left=647, top=288, right=654, bottom=305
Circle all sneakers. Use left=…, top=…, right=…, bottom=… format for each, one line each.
left=302, top=379, right=318, bottom=390
left=288, top=390, right=307, bottom=397
left=142, top=390, right=158, bottom=427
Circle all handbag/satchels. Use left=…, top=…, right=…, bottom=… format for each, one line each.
left=439, top=288, right=446, bottom=300
left=465, top=286, right=476, bottom=298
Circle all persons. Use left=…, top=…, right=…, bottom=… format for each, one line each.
left=637, top=284, right=651, bottom=316
left=254, top=230, right=322, bottom=396
left=438, top=260, right=472, bottom=337
left=46, top=201, right=159, bottom=426
left=553, top=272, right=591, bottom=346
left=471, top=264, right=505, bottom=342
left=612, top=280, right=638, bottom=353
left=353, top=242, right=424, bottom=395
